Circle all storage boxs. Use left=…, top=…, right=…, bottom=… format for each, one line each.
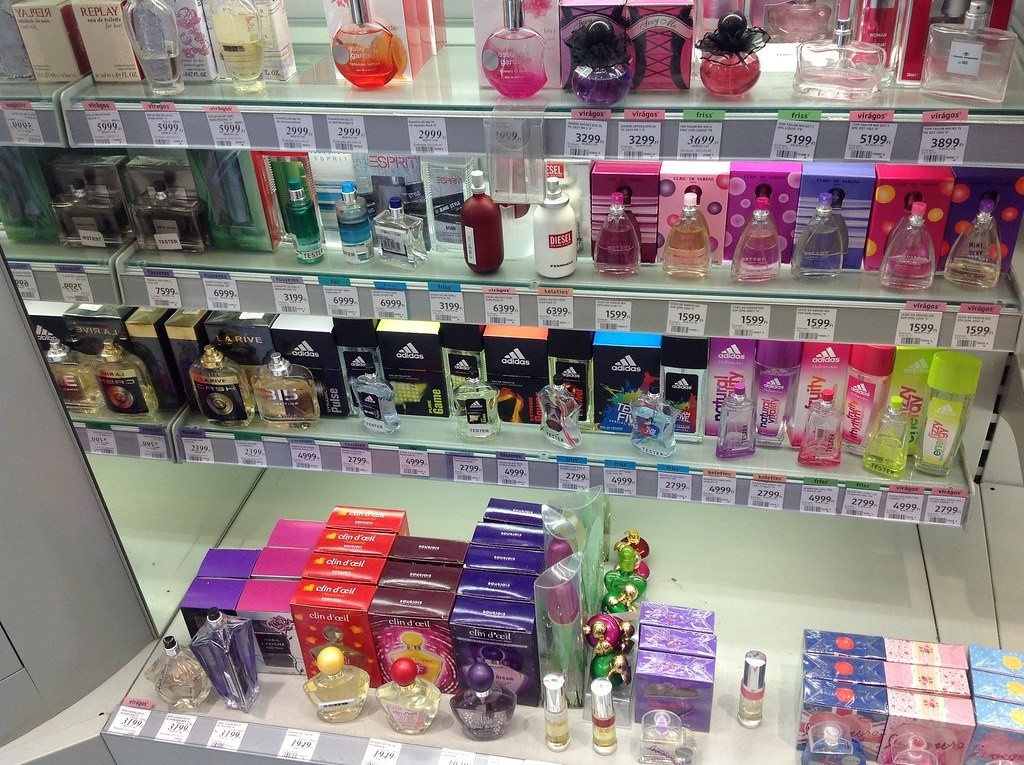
left=235, top=578, right=308, bottom=675
left=326, top=506, right=410, bottom=536
left=375, top=561, right=463, bottom=592
left=301, top=552, right=387, bottom=585
left=387, top=536, right=470, bottom=568
left=251, top=547, right=314, bottom=582
left=448, top=594, right=548, bottom=708
left=0, top=0, right=1012, bottom=439
left=313, top=529, right=397, bottom=558
left=289, top=578, right=384, bottom=690
left=180, top=548, right=262, bottom=640
left=471, top=521, right=545, bottom=552
left=367, top=587, right=459, bottom=696
left=463, top=543, right=546, bottom=576
left=635, top=650, right=715, bottom=732
left=483, top=497, right=564, bottom=527
left=268, top=517, right=326, bottom=551
left=638, top=602, right=717, bottom=659
left=795, top=628, right=1024, bottom=765
left=457, top=569, right=540, bottom=603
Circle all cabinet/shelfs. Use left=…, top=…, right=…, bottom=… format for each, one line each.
left=0, top=0, right=1024, bottom=765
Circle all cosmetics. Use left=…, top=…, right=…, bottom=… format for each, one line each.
left=1, top=0, right=1023, bottom=765
